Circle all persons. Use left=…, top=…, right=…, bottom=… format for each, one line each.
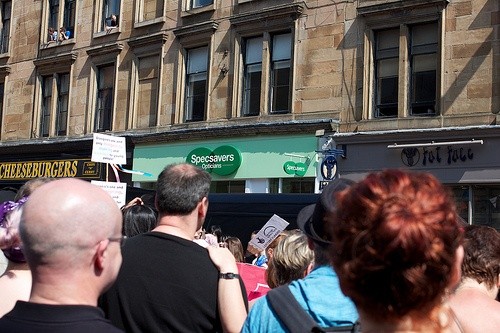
left=120, top=193, right=159, bottom=238
left=207, top=177, right=356, bottom=333
left=0, top=177, right=59, bottom=318
left=57, top=27, right=73, bottom=41
left=0, top=178, right=127, bottom=333
left=48, top=27, right=57, bottom=44
left=105, top=14, right=119, bottom=30
left=98, top=164, right=248, bottom=333
left=197, top=225, right=313, bottom=288
left=327, top=168, right=500, bottom=333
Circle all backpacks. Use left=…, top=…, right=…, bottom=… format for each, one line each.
left=266, top=286, right=360, bottom=333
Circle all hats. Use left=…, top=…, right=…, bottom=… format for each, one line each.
left=297, top=177, right=356, bottom=245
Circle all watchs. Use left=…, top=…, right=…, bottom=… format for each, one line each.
left=220, top=273, right=239, bottom=279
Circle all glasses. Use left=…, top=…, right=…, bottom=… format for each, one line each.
left=109, top=235, right=128, bottom=247
